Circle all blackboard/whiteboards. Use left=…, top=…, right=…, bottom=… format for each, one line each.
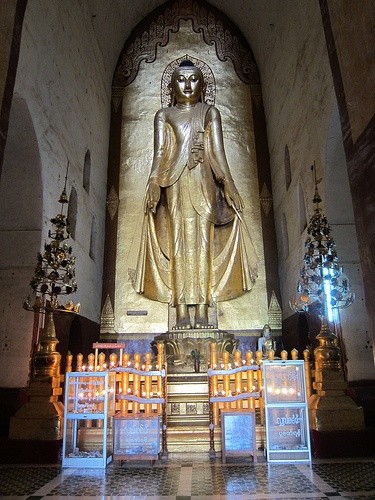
left=222, top=413, right=257, bottom=455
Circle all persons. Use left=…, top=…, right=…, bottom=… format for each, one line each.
left=257, top=325, right=277, bottom=359
left=132, top=60, right=260, bottom=331
